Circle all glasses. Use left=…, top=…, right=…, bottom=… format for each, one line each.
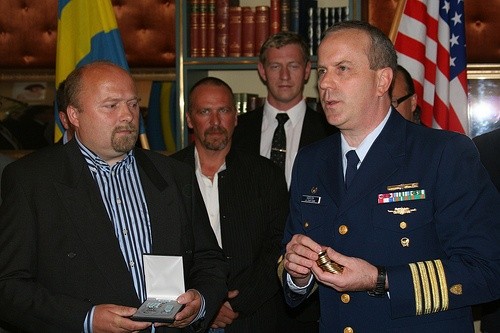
left=391, top=90, right=415, bottom=110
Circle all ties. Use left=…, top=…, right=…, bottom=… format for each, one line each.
left=343, top=148, right=360, bottom=189
left=269, top=112, right=290, bottom=175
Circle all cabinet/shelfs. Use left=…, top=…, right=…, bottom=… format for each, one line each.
left=0, top=0, right=500, bottom=157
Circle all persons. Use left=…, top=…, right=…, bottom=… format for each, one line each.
left=167, top=77, right=290, bottom=333
left=17, top=84, right=46, bottom=102
left=270, top=20, right=500, bottom=333
left=0, top=61, right=234, bottom=333
left=230, top=33, right=341, bottom=193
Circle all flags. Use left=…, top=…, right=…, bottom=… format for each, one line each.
left=393, top=0, right=473, bottom=140
left=55, top=0, right=150, bottom=151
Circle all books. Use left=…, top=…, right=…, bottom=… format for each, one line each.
left=190, top=0, right=350, bottom=116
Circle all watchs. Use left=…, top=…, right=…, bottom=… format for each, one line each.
left=367, top=263, right=388, bottom=299
